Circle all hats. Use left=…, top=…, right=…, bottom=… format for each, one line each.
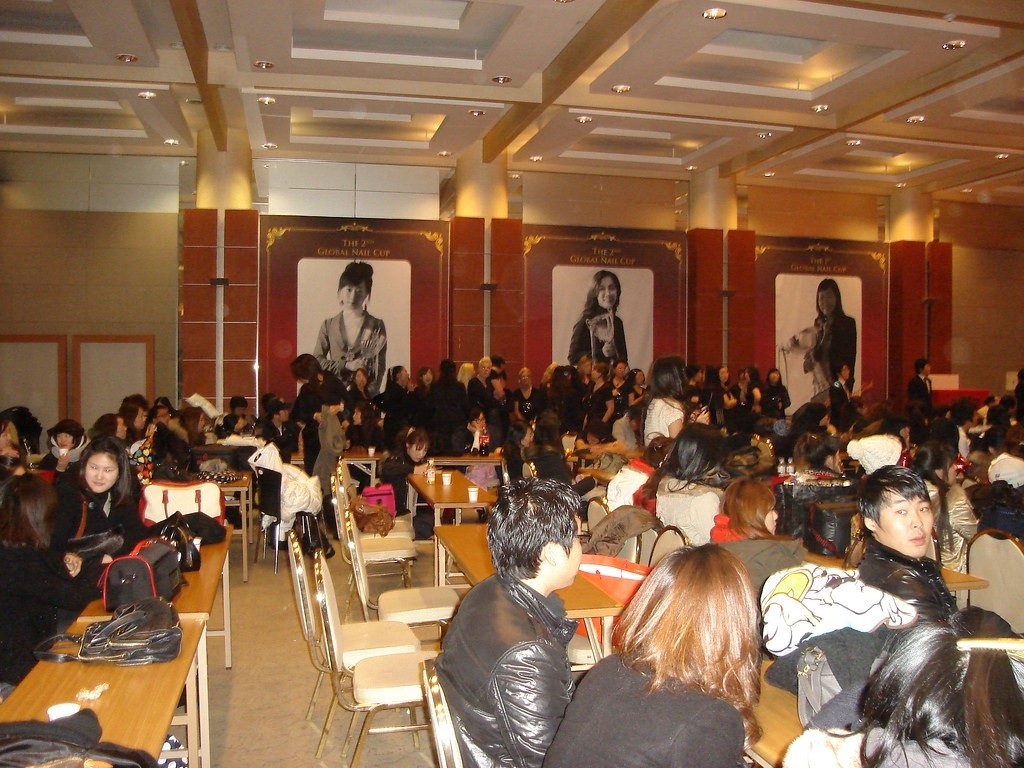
left=987, top=453, right=1024, bottom=489
left=847, top=434, right=903, bottom=476
left=265, top=400, right=287, bottom=416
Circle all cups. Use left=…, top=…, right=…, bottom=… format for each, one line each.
left=368, top=448, right=375, bottom=456
left=59, top=449, right=68, bottom=457
left=442, top=474, right=451, bottom=486
left=47, top=703, right=80, bottom=722
left=468, top=487, right=478, bottom=503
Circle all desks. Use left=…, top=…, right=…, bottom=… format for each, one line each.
left=746, top=660, right=805, bottom=768
left=406, top=469, right=496, bottom=587
left=433, top=523, right=624, bottom=672
left=428, top=456, right=503, bottom=465
left=0, top=444, right=386, bottom=768
left=777, top=535, right=989, bottom=591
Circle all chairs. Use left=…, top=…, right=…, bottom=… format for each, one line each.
left=521, top=431, right=692, bottom=569
left=965, top=526, right=1024, bottom=636
left=751, top=434, right=775, bottom=461
left=258, top=455, right=466, bottom=768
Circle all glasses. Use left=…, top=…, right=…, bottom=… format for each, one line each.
left=472, top=417, right=486, bottom=424
left=481, top=367, right=491, bottom=371
left=574, top=529, right=593, bottom=545
left=521, top=376, right=529, bottom=379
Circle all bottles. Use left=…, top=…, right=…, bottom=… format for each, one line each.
left=478, top=422, right=489, bottom=457
left=426, top=459, right=436, bottom=484
left=777, top=457, right=795, bottom=476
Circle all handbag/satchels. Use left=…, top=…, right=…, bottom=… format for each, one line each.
left=573, top=554, right=654, bottom=646
left=0, top=708, right=159, bottom=768
left=802, top=498, right=858, bottom=557
left=349, top=477, right=395, bottom=537
left=33, top=511, right=228, bottom=668
left=764, top=624, right=886, bottom=726
left=319, top=404, right=345, bottom=457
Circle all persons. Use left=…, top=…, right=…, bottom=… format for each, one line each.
left=314, top=262, right=389, bottom=398
left=0, top=354, right=1024, bottom=768
left=803, top=279, right=857, bottom=392
left=567, top=270, right=628, bottom=367
